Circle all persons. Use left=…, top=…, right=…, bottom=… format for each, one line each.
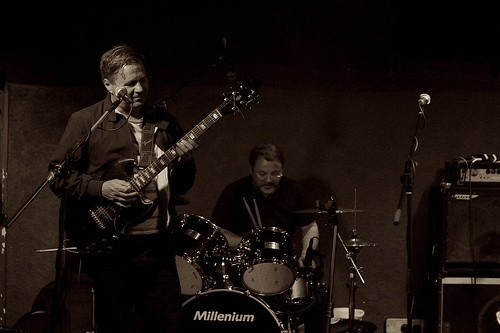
left=45, top=45, right=202, bottom=289
left=211, top=143, right=322, bottom=272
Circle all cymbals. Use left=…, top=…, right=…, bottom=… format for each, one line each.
left=293, top=207, right=364, bottom=214
left=325, top=238, right=388, bottom=250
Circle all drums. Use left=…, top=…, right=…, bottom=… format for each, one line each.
left=180, top=288, right=287, bottom=333
left=241, top=226, right=297, bottom=297
left=270, top=271, right=316, bottom=308
left=171, top=212, right=230, bottom=294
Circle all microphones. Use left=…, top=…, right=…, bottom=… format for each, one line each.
left=115, top=86, right=133, bottom=103
left=418, top=93, right=431, bottom=104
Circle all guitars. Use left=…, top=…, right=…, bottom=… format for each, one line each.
left=64, top=79, right=260, bottom=256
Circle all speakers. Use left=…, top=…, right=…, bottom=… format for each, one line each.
left=428, top=275, right=500, bottom=333
left=431, top=186, right=500, bottom=272
